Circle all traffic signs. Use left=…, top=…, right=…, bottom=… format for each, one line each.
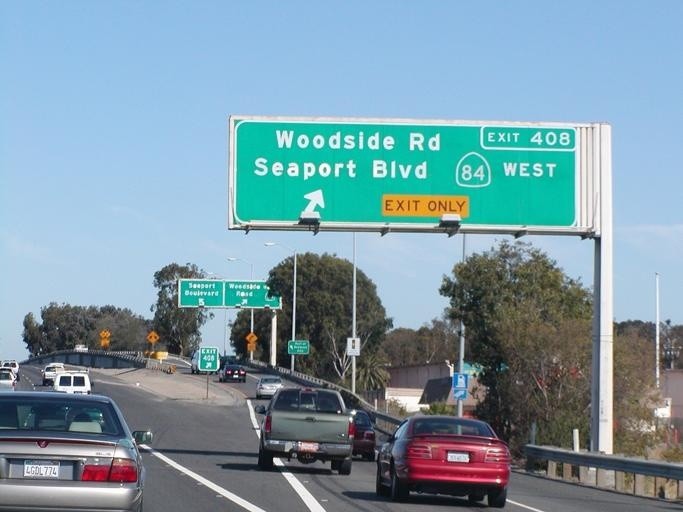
left=229, top=115, right=577, bottom=234
left=177, top=278, right=281, bottom=310
left=288, top=340, right=309, bottom=354
left=197, top=348, right=220, bottom=371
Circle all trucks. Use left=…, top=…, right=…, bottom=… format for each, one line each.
left=253, top=387, right=352, bottom=476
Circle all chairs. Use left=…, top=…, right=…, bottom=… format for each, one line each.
left=417, top=425, right=432, bottom=433
left=68, top=421, right=102, bottom=433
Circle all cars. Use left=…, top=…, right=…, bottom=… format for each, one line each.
left=1, top=390, right=152, bottom=512
left=0, top=359, right=20, bottom=390
left=256, top=377, right=284, bottom=396
left=347, top=408, right=375, bottom=460
left=192, top=347, right=248, bottom=383
left=376, top=417, right=516, bottom=508
left=40, top=362, right=92, bottom=394
